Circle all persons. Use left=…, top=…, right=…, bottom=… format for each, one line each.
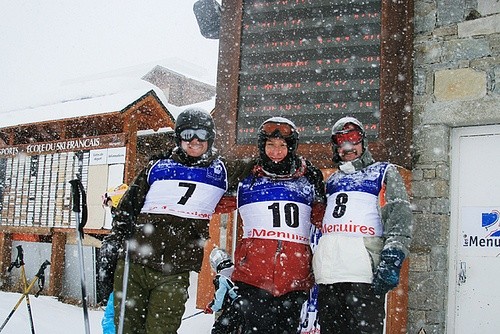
left=209, top=118, right=327, bottom=334
left=99, top=108, right=251, bottom=333
left=312, top=117, right=413, bottom=334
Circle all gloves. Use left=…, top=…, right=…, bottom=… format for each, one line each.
left=99, top=233, right=123, bottom=264
left=371, top=248, right=406, bottom=296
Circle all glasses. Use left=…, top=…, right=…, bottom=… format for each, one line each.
left=176, top=126, right=214, bottom=141
left=332, top=128, right=365, bottom=147
left=257, top=120, right=296, bottom=138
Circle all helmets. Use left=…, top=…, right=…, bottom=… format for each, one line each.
left=175, top=106, right=216, bottom=147
left=257, top=116, right=300, bottom=156
